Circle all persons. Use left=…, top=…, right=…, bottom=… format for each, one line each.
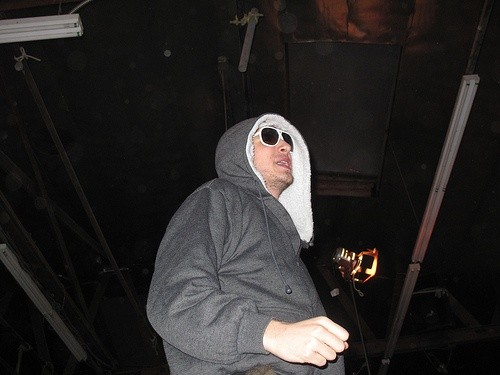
left=147, top=112, right=350, bottom=375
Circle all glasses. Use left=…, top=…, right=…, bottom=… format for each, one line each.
left=252, top=126, right=293, bottom=152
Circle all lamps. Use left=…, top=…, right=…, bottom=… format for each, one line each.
left=0, top=13, right=84, bottom=44
left=0, top=243, right=88, bottom=363
left=379, top=75, right=479, bottom=375
